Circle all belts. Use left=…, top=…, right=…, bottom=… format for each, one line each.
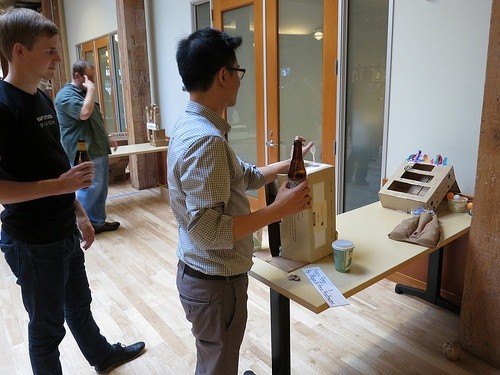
left=178, top=260, right=248, bottom=280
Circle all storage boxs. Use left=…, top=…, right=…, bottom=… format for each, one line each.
left=252, top=160, right=337, bottom=273
left=378, top=157, right=461, bottom=213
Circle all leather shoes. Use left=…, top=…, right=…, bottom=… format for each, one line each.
left=94, top=341, right=145, bottom=375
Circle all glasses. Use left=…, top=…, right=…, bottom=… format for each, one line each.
left=227, top=67, right=246, bottom=80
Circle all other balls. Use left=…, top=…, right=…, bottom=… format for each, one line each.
left=453, top=195, right=460, bottom=200
left=467, top=202, right=474, bottom=209
left=447, top=192, right=454, bottom=200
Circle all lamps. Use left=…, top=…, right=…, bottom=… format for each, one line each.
left=313, top=29, right=323, bottom=40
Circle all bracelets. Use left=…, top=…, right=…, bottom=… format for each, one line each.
left=108, top=134, right=112, bottom=137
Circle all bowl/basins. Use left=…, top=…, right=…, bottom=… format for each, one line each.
left=448, top=196, right=468, bottom=213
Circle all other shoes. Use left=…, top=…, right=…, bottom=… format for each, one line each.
left=93, top=221, right=120, bottom=235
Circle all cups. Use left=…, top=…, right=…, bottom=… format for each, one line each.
left=332, top=239, right=355, bottom=273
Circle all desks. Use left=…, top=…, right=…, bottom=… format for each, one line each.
left=245, top=199, right=471, bottom=375
left=108, top=143, right=168, bottom=158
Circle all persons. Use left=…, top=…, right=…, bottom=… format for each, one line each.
left=55, top=60, right=120, bottom=234
left=0, top=8, right=145, bottom=375
left=167, top=27, right=315, bottom=375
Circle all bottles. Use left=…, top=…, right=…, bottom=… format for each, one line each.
left=287, top=140, right=306, bottom=189
left=74, top=138, right=92, bottom=189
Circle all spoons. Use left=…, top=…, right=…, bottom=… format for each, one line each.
left=310, top=145, right=316, bottom=162
